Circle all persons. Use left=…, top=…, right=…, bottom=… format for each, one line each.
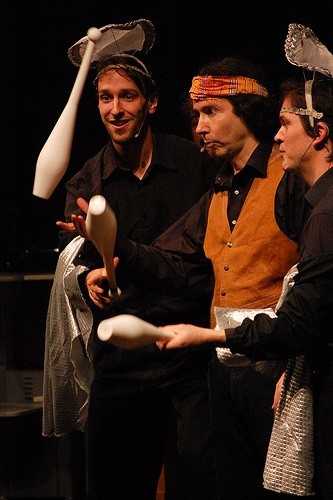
left=56, top=55, right=310, bottom=500
left=52, top=52, right=229, bottom=500
left=154, top=77, right=333, bottom=500
